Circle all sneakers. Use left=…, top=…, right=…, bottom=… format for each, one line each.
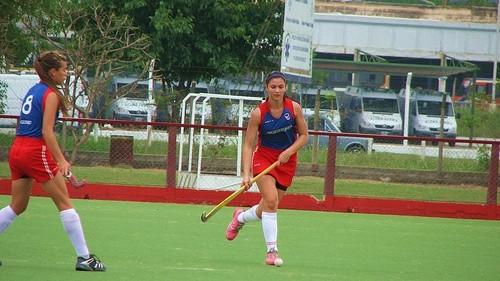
left=266, top=251, right=279, bottom=264
left=75, top=254, right=106, bottom=271
left=225, top=208, right=243, bottom=241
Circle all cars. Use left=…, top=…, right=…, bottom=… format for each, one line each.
left=302, top=112, right=375, bottom=156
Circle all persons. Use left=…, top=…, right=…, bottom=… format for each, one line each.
left=0, top=50, right=106, bottom=272
left=226, top=70, right=308, bottom=265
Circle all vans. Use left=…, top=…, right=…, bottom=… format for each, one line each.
left=167, top=80, right=213, bottom=123
left=399, top=86, right=461, bottom=147
left=220, top=84, right=266, bottom=124
left=291, top=87, right=342, bottom=134
left=340, top=85, right=403, bottom=144
left=86, top=76, right=157, bottom=129
left=0, top=74, right=88, bottom=131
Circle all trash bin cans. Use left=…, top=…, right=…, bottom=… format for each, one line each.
left=109, top=135, right=134, bottom=167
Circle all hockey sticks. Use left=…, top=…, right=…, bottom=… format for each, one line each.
left=66, top=171, right=88, bottom=188
left=200, top=160, right=279, bottom=222
